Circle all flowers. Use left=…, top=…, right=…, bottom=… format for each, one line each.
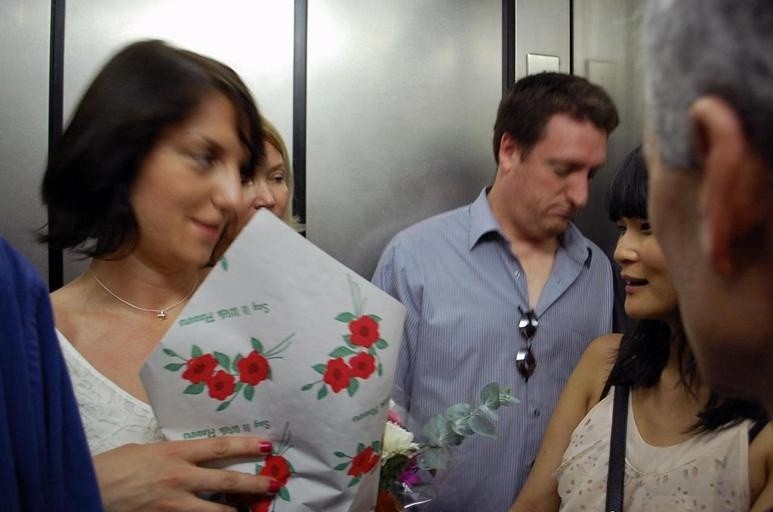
left=382, top=373, right=524, bottom=491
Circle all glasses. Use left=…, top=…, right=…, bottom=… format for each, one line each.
left=515, top=305, right=539, bottom=383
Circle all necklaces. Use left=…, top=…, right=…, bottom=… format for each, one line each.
left=88, top=266, right=200, bottom=317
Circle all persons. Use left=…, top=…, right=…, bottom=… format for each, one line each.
left=642, top=0, right=773, bottom=406
left=370, top=71, right=619, bottom=512
left=1, top=236, right=106, bottom=511
left=510, top=143, right=772, bottom=512
left=205, top=117, right=306, bottom=267
left=39, top=39, right=279, bottom=512
